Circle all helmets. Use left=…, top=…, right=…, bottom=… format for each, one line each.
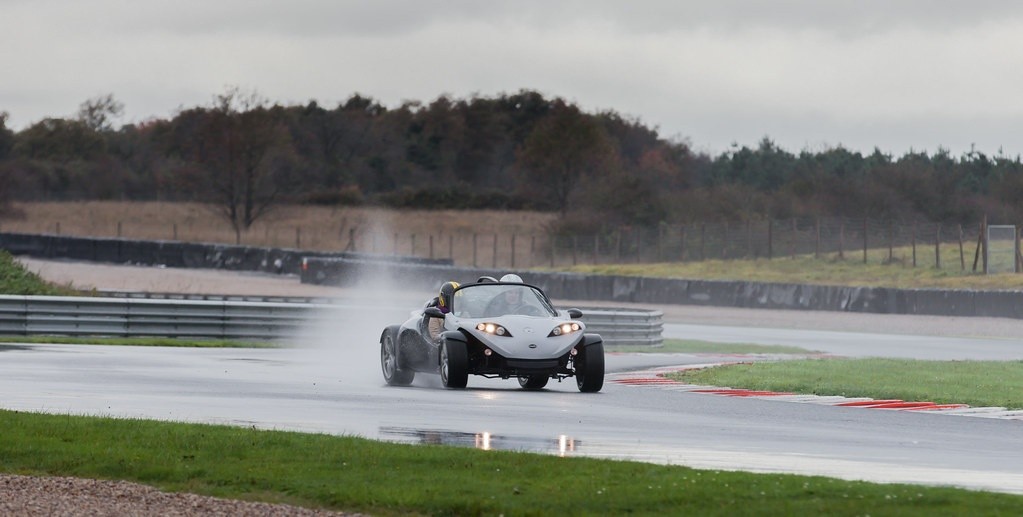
left=438, top=281, right=463, bottom=307
left=499, top=274, right=523, bottom=290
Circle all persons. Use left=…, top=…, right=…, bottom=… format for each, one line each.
left=427, top=280, right=482, bottom=344
left=489, top=273, right=547, bottom=318
